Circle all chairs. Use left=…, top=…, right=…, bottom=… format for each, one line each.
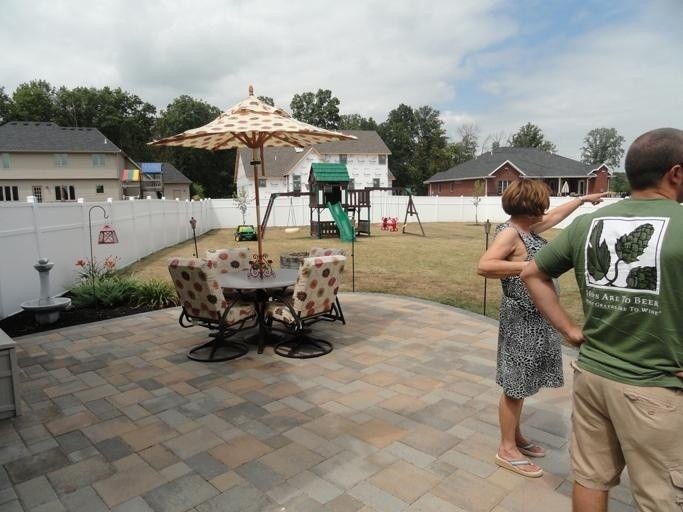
left=285, top=247, right=346, bottom=325
left=265, top=255, right=346, bottom=358
left=168, top=258, right=258, bottom=363
left=206, top=248, right=257, bottom=331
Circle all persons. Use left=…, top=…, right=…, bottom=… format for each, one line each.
left=476, top=178, right=610, bottom=479
left=520, top=129, right=683, bottom=512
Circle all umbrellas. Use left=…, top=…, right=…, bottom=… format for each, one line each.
left=147, top=84, right=357, bottom=278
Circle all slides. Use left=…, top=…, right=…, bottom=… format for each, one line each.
left=327, top=202, right=356, bottom=241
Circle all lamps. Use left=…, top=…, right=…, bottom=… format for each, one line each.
left=98, top=217, right=120, bottom=246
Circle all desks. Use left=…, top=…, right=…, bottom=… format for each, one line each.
left=218, top=269, right=299, bottom=354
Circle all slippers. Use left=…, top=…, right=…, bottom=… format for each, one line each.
left=495, top=453, right=543, bottom=478
left=516, top=442, right=545, bottom=457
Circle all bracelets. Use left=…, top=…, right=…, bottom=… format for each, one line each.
left=578, top=196, right=584, bottom=206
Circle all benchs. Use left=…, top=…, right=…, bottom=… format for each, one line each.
left=234, top=225, right=254, bottom=241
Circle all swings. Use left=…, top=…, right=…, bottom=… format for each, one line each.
left=285, top=197, right=300, bottom=233
left=379, top=191, right=390, bottom=231
left=391, top=190, right=400, bottom=231
